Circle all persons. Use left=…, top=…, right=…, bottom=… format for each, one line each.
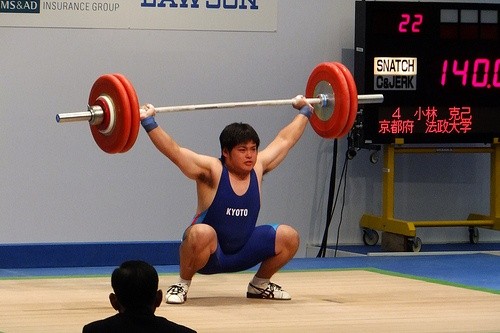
left=83, top=260, right=198, bottom=333
left=139, top=94, right=315, bottom=304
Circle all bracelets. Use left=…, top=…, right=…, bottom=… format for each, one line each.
left=140, top=116, right=159, bottom=133
left=298, top=105, right=315, bottom=119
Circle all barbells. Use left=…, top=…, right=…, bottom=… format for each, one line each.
left=56, top=62, right=384, bottom=153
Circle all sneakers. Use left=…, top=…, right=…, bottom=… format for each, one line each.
left=164, top=283, right=188, bottom=303
left=247, top=281, right=292, bottom=300
